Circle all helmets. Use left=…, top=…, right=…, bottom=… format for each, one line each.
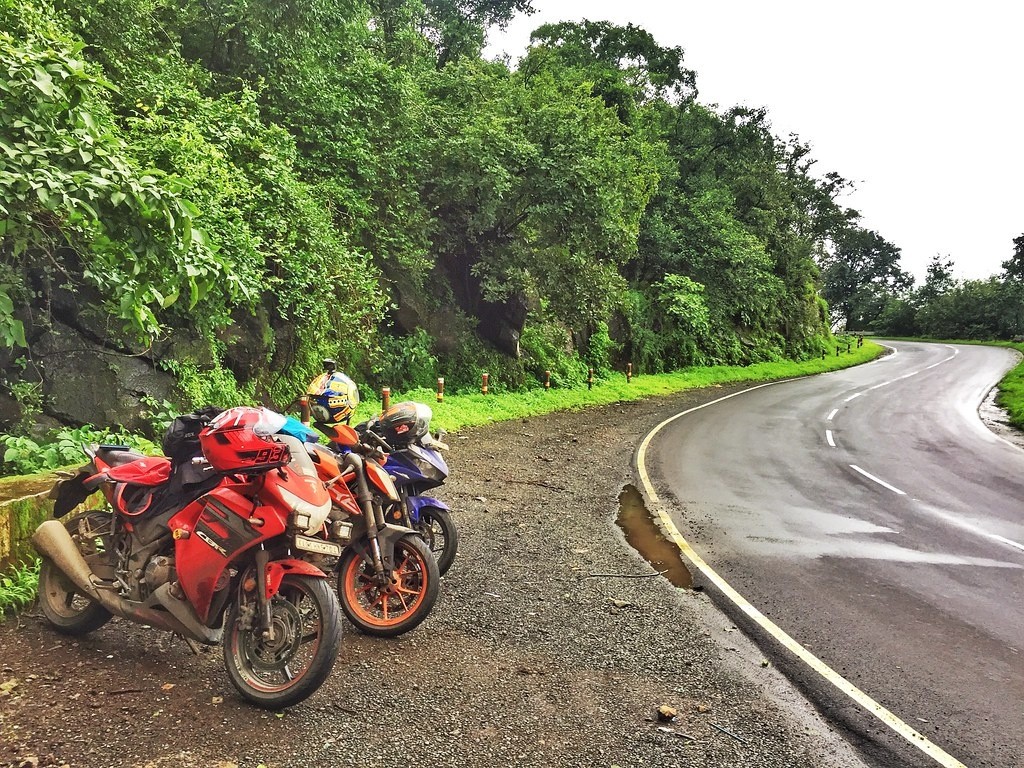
left=306, top=371, right=360, bottom=424
left=197, top=406, right=292, bottom=475
left=380, top=403, right=428, bottom=445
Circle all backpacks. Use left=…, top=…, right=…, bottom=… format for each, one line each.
left=83, top=456, right=172, bottom=516
left=162, top=405, right=227, bottom=465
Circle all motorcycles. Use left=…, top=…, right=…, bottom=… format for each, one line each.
left=31, top=395, right=459, bottom=711
